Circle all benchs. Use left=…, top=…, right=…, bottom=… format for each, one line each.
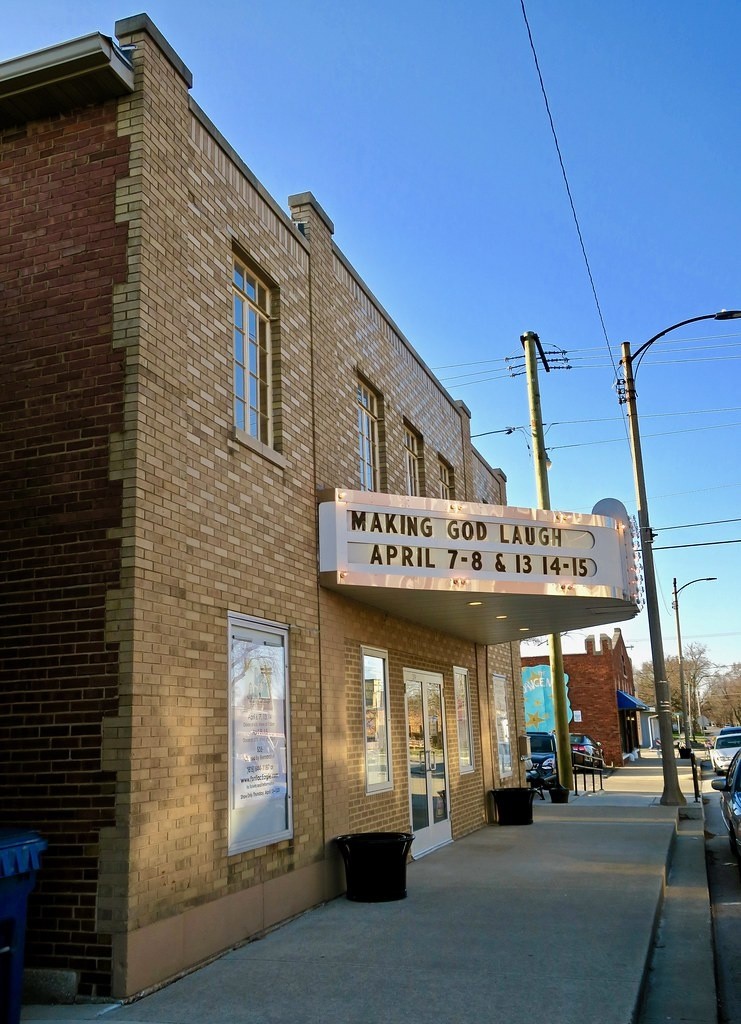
left=526, top=768, right=558, bottom=801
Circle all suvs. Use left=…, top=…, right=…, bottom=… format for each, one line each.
left=525, top=731, right=559, bottom=789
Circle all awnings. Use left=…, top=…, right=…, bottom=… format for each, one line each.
left=617, top=690, right=650, bottom=711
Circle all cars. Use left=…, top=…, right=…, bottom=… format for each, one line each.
left=569, top=733, right=604, bottom=772
left=710, top=747, right=741, bottom=857
left=709, top=734, right=741, bottom=776
left=720, top=726, right=741, bottom=735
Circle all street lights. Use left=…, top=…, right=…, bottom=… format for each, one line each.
left=615, top=309, right=741, bottom=807
left=670, top=576, right=719, bottom=751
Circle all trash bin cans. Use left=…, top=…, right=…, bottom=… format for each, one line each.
left=0, top=829, right=50, bottom=1024
